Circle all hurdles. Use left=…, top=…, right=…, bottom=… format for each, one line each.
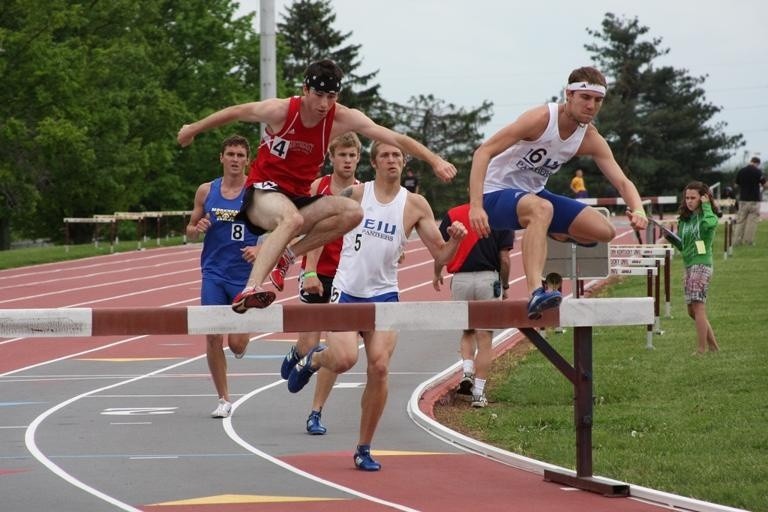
left=1, top=297, right=655, bottom=497
left=63, top=210, right=192, bottom=254
left=572, top=195, right=678, bottom=299
left=609, top=243, right=675, bottom=348
left=654, top=213, right=738, bottom=261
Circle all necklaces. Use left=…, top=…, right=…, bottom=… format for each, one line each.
left=563, top=103, right=585, bottom=133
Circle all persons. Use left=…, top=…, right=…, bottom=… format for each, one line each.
left=186, top=135, right=263, bottom=418
left=536, top=273, right=565, bottom=339
left=401, top=165, right=419, bottom=193
left=729, top=157, right=768, bottom=247
left=287, top=136, right=468, bottom=471
left=433, top=178, right=515, bottom=407
left=176, top=60, right=458, bottom=313
left=569, top=169, right=589, bottom=198
left=468, top=66, right=648, bottom=319
left=280, top=132, right=365, bottom=380
left=675, top=182, right=723, bottom=359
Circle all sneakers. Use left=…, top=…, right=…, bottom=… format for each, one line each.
left=452, top=370, right=494, bottom=409
left=279, top=340, right=328, bottom=395
left=208, top=393, right=235, bottom=421
left=306, top=406, right=330, bottom=435
left=350, top=443, right=383, bottom=474
left=231, top=282, right=277, bottom=317
left=269, top=245, right=299, bottom=293
left=524, top=283, right=563, bottom=322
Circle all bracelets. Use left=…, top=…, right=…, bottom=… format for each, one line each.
left=303, top=272, right=318, bottom=278
left=633, top=210, right=645, bottom=218
left=503, top=284, right=510, bottom=289
